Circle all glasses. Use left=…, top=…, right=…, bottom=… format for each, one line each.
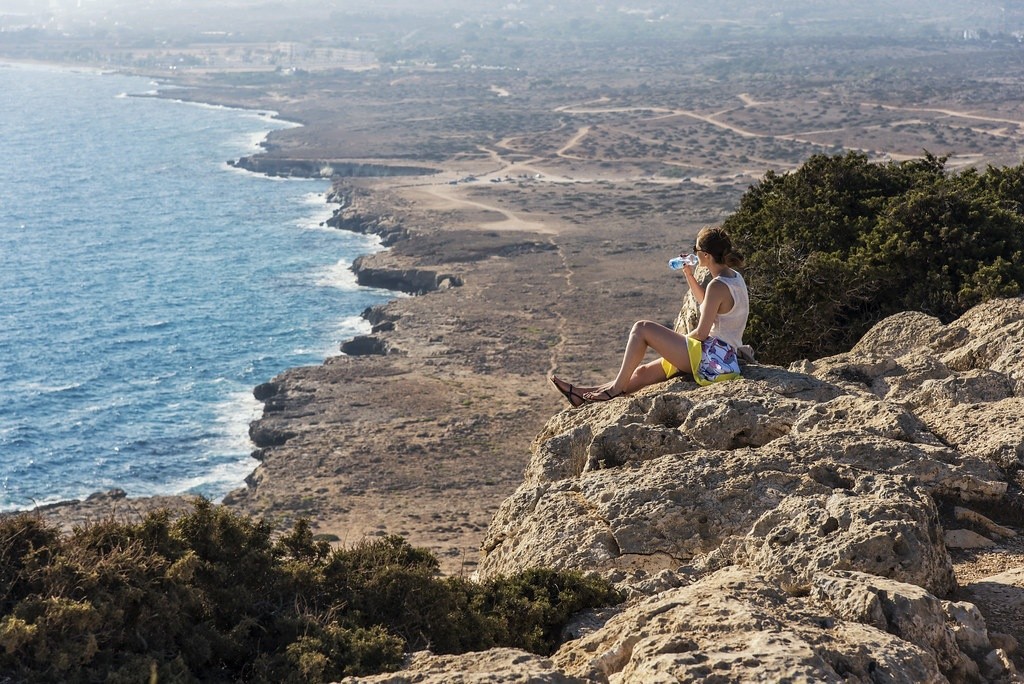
left=692, top=246, right=704, bottom=256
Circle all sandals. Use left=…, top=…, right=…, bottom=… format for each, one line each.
left=550, top=375, right=585, bottom=406
left=582, top=390, right=625, bottom=401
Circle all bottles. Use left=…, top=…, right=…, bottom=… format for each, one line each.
left=668, top=254, right=699, bottom=270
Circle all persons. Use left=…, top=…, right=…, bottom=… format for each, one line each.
left=551, top=228, right=749, bottom=407
